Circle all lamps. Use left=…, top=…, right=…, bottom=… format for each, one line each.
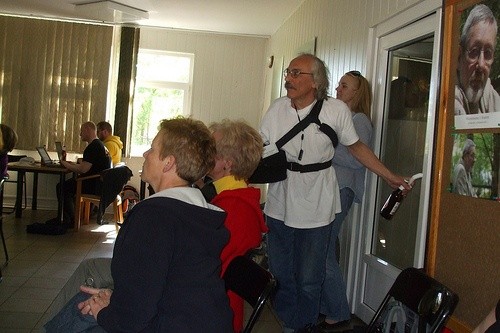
left=76, top=1, right=149, bottom=24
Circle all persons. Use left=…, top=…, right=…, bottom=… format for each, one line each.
left=454, top=4, right=499, bottom=116
left=74, top=121, right=125, bottom=221
left=39, top=117, right=238, bottom=333
left=312, top=69, right=379, bottom=329
left=0, top=124, right=17, bottom=179
left=452, top=136, right=480, bottom=198
left=54, top=121, right=112, bottom=228
left=262, top=52, right=419, bottom=332
left=47, top=117, right=268, bottom=333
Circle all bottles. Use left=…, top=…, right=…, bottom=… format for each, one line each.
left=380, top=180, right=409, bottom=220
left=62, top=146, right=66, bottom=159
left=41, top=145, right=46, bottom=166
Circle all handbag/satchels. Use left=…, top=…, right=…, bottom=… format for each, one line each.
left=26, top=222, right=67, bottom=234
left=248, top=150, right=288, bottom=184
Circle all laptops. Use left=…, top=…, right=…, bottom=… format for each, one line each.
left=36, top=147, right=61, bottom=168
left=56, top=142, right=76, bottom=165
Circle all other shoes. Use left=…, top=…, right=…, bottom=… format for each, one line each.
left=90, top=203, right=98, bottom=216
left=318, top=319, right=351, bottom=330
left=46, top=217, right=74, bottom=228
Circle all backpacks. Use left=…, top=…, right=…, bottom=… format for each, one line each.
left=120, top=185, right=140, bottom=218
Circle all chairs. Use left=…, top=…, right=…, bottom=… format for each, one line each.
left=0, top=177, right=10, bottom=280
left=223, top=254, right=277, bottom=333
left=367, top=267, right=459, bottom=333
left=73, top=165, right=133, bottom=231
left=2, top=155, right=27, bottom=213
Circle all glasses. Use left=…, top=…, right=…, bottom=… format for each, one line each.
left=284, top=69, right=314, bottom=78
left=351, top=71, right=360, bottom=77
left=464, top=47, right=493, bottom=58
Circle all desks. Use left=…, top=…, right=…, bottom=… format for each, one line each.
left=7, top=161, right=70, bottom=222
